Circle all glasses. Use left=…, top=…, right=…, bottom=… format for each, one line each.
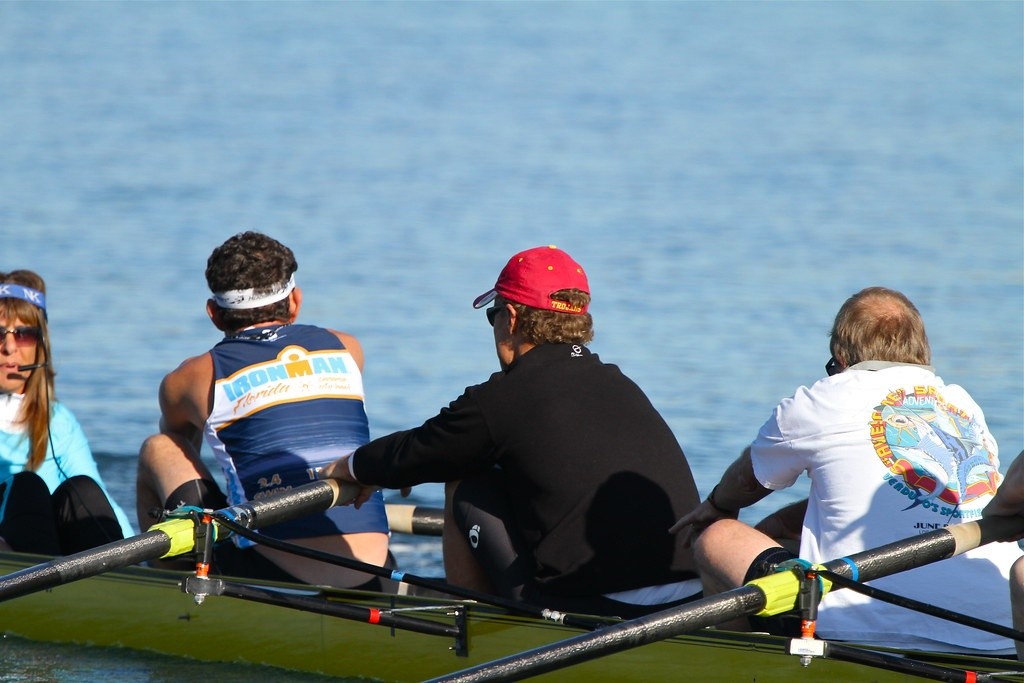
left=0, top=327, right=39, bottom=347
left=487, top=304, right=523, bottom=327
left=825, top=355, right=837, bottom=377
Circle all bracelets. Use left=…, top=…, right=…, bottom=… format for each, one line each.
left=707, top=485, right=741, bottom=513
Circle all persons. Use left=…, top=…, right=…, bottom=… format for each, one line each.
left=135, top=230, right=400, bottom=602
left=0, top=270, right=137, bottom=574
left=670, top=288, right=1024, bottom=658
left=981, top=450, right=1024, bottom=676
left=323, top=245, right=704, bottom=631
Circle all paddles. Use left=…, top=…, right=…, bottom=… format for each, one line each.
left=412, top=509, right=1024, bottom=683
left=0, top=474, right=387, bottom=605
left=386, top=498, right=451, bottom=536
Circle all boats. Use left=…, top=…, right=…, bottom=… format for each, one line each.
left=0, top=541, right=1024, bottom=683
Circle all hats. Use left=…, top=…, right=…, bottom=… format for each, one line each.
left=472, top=245, right=591, bottom=315
